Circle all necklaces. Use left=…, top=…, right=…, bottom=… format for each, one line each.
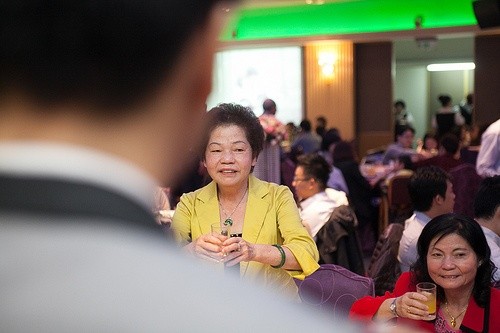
left=216, top=188, right=248, bottom=227
left=443, top=302, right=470, bottom=327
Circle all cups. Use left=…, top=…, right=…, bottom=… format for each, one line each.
left=210, top=220, right=231, bottom=255
left=416, top=282, right=437, bottom=320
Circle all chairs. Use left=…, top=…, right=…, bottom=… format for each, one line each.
left=294, top=150, right=478, bottom=318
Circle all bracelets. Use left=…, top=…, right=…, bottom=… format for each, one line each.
left=269, top=244, right=285, bottom=269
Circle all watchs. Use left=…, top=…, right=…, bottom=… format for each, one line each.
left=389, top=298, right=402, bottom=318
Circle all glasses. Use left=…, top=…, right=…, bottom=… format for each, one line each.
left=293, top=176, right=316, bottom=183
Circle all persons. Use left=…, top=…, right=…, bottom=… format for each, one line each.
left=471, top=111, right=500, bottom=282
left=397, top=166, right=456, bottom=274
left=382, top=127, right=426, bottom=162
left=286, top=117, right=326, bottom=152
left=170, top=103, right=319, bottom=307
left=394, top=102, right=415, bottom=141
left=0, top=0, right=416, bottom=333
left=330, top=143, right=367, bottom=195
left=417, top=131, right=439, bottom=150
left=431, top=96, right=465, bottom=144
left=292, top=153, right=350, bottom=237
left=350, top=214, right=500, bottom=333
left=463, top=95, right=475, bottom=126
left=384, top=155, right=415, bottom=186
left=254, top=99, right=290, bottom=139
